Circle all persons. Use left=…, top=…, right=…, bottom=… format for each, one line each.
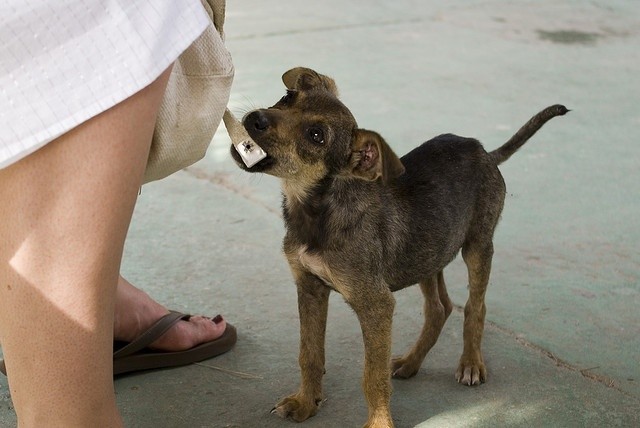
left=0, top=1, right=238, bottom=428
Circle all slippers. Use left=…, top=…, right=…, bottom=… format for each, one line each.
left=0, top=310, right=237, bottom=375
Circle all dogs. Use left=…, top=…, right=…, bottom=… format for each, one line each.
left=230, top=65, right=571, bottom=428
